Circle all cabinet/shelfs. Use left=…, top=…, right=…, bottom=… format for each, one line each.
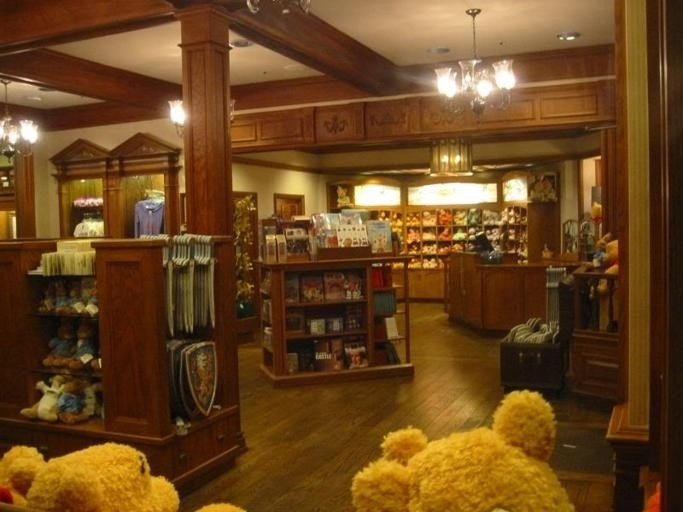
left=426, top=132, right=476, bottom=179
left=253, top=211, right=414, bottom=389
left=25, top=273, right=102, bottom=377
left=343, top=200, right=528, bottom=337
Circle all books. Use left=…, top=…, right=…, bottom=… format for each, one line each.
left=285, top=268, right=401, bottom=374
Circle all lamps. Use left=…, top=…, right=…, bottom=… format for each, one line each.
left=433, top=6, right=518, bottom=129
left=0, top=78, right=43, bottom=162
left=168, top=86, right=239, bottom=141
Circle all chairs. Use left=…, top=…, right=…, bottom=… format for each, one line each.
left=498, top=277, right=569, bottom=397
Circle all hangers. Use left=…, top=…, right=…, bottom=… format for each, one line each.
left=142, top=187, right=164, bottom=204
left=80, top=210, right=102, bottom=223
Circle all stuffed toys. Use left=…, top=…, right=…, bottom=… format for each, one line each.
left=19, top=276, right=103, bottom=425
left=378, top=207, right=528, bottom=270
left=0, top=441, right=246, bottom=512
left=588, top=232, right=618, bottom=300
left=350, top=387, right=576, bottom=511
